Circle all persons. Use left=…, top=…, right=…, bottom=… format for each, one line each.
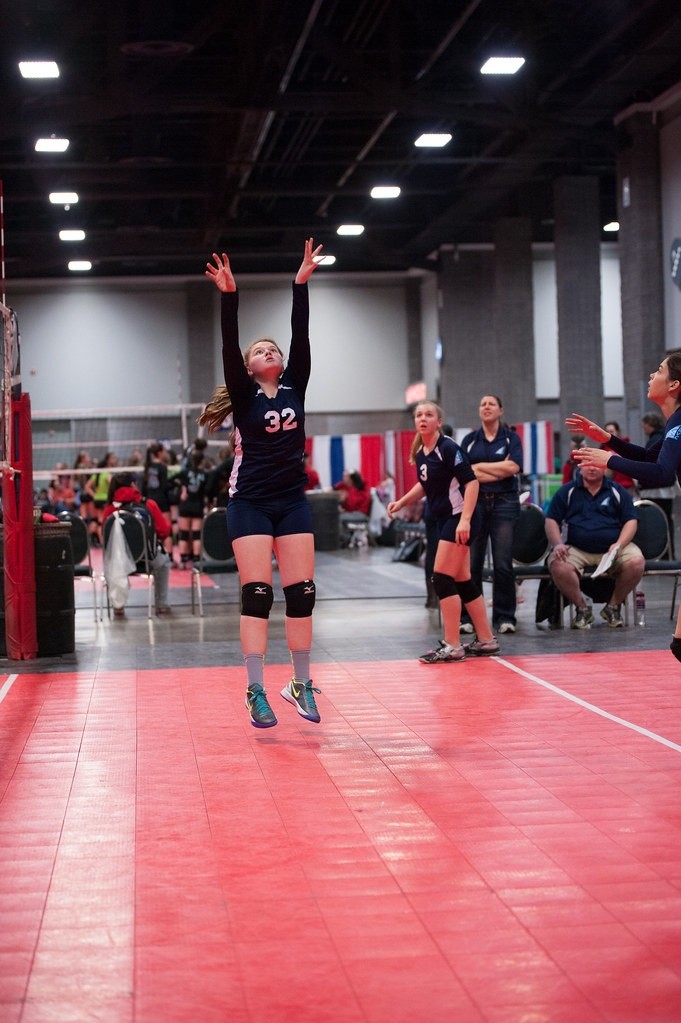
left=385, top=400, right=503, bottom=664
left=636, top=410, right=681, bottom=561
left=194, top=237, right=327, bottom=729
left=32, top=437, right=231, bottom=571
left=562, top=420, right=640, bottom=504
left=458, top=395, right=525, bottom=635
left=302, top=451, right=396, bottom=549
left=564, top=352, right=681, bottom=663
left=100, top=471, right=173, bottom=620
left=544, top=459, right=645, bottom=630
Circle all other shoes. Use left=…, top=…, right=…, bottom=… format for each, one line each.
left=460, top=623, right=473, bottom=634
left=114, top=608, right=125, bottom=617
left=156, top=607, right=172, bottom=618
left=498, top=623, right=515, bottom=634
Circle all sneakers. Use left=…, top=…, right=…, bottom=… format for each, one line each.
left=464, top=634, right=501, bottom=656
left=245, top=683, right=277, bottom=728
left=280, top=677, right=320, bottom=723
left=600, top=605, right=623, bottom=628
left=574, top=606, right=594, bottom=628
left=418, top=640, right=466, bottom=663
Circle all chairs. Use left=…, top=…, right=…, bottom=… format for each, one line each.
left=58, top=507, right=235, bottom=622
left=486, top=500, right=681, bottom=627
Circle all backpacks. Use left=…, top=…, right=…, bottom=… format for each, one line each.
left=103, top=506, right=158, bottom=574
left=186, top=469, right=206, bottom=495
